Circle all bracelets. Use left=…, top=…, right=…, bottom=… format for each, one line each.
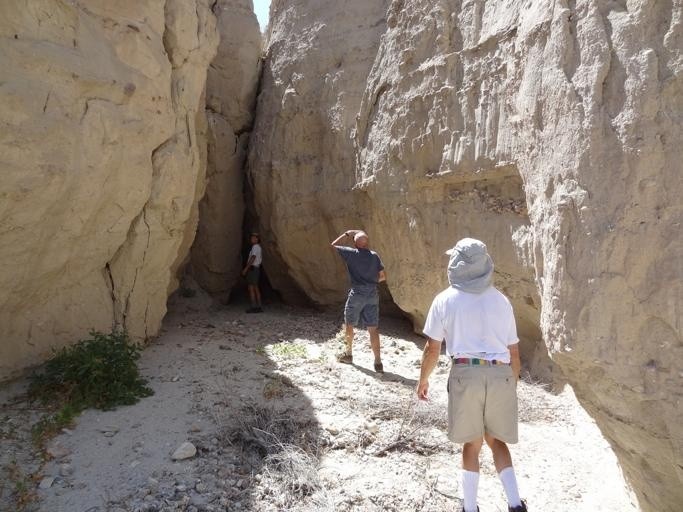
left=345, top=232, right=349, bottom=236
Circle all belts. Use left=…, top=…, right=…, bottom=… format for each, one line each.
left=452, top=358, right=503, bottom=365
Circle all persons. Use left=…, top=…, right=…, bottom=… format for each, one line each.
left=331, top=229, right=384, bottom=372
left=417, top=237, right=527, bottom=511
left=240, top=233, right=263, bottom=314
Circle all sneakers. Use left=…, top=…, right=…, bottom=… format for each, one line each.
left=462, top=505, right=479, bottom=512
left=508, top=500, right=527, bottom=512
left=246, top=306, right=263, bottom=313
left=374, top=363, right=383, bottom=373
left=334, top=352, right=352, bottom=364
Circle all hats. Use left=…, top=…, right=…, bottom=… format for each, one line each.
left=445, top=247, right=454, bottom=255
left=248, top=233, right=263, bottom=244
